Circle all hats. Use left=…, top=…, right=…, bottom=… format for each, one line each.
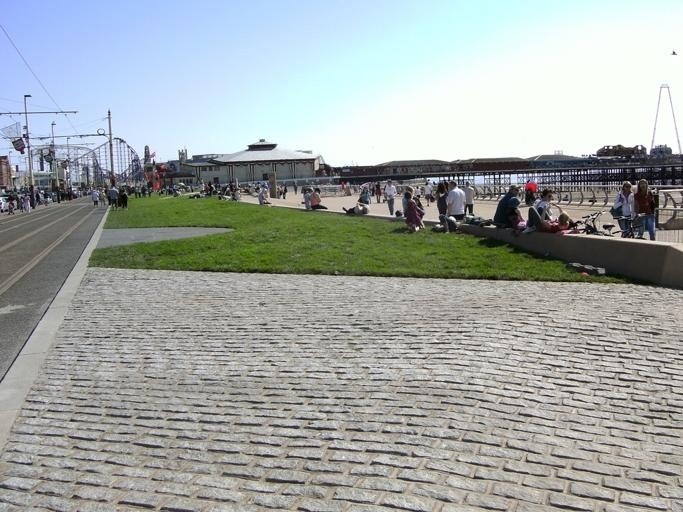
left=508, top=184, right=521, bottom=190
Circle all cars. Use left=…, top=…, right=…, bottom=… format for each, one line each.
left=0, top=184, right=93, bottom=214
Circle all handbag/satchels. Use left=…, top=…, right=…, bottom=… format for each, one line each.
left=608, top=194, right=622, bottom=217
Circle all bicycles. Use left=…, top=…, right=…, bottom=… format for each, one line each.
left=599, top=214, right=651, bottom=241
left=573, top=210, right=609, bottom=235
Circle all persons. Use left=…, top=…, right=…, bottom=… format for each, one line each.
left=4, top=182, right=148, bottom=217
left=632, top=178, right=658, bottom=242
left=304, top=190, right=311, bottom=210
left=310, top=188, right=328, bottom=210
left=293, top=184, right=299, bottom=194
left=337, top=179, right=587, bottom=236
left=159, top=179, right=287, bottom=206
left=613, top=181, right=635, bottom=239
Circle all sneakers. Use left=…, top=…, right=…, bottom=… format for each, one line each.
left=522, top=225, right=536, bottom=233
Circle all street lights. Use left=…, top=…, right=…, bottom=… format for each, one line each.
left=66, top=136, right=72, bottom=190
left=50, top=119, right=60, bottom=194
left=23, top=94, right=35, bottom=208
